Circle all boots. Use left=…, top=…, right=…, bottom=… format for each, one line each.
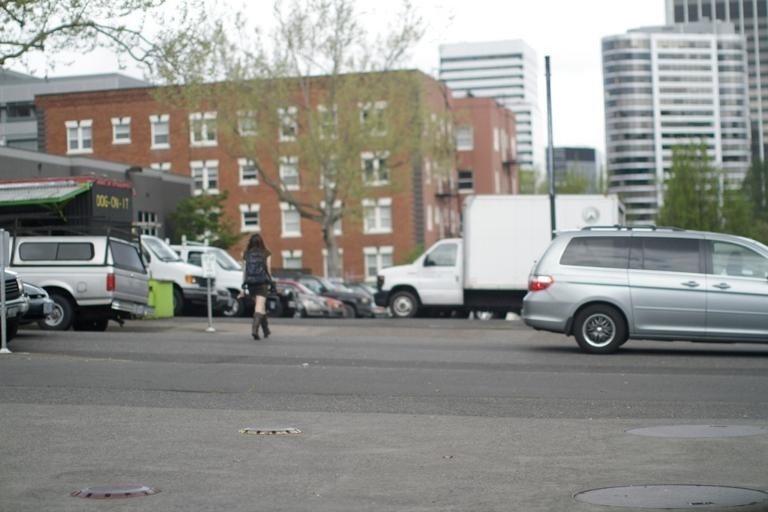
left=260, top=314, right=271, bottom=338
left=250, top=311, right=264, bottom=341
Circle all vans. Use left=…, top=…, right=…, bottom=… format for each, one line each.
left=515, top=223, right=767, bottom=356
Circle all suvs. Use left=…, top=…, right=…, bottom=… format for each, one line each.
left=136, top=234, right=216, bottom=316
left=8, top=234, right=151, bottom=330
left=169, top=244, right=258, bottom=317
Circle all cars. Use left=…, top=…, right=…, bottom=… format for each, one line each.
left=269, top=274, right=381, bottom=319
left=2, top=269, right=55, bottom=343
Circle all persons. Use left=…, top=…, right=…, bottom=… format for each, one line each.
left=235, top=232, right=277, bottom=340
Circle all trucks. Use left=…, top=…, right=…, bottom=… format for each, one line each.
left=374, top=193, right=625, bottom=323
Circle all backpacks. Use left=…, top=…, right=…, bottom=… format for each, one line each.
left=244, top=246, right=267, bottom=286
